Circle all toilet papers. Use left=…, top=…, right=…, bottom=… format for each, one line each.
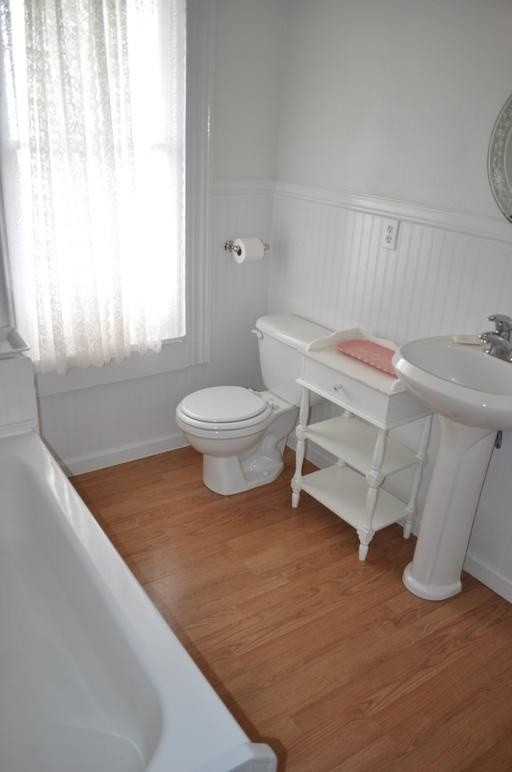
left=232, top=237, right=264, bottom=265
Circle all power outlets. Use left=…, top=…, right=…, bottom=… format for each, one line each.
left=380, top=219, right=401, bottom=250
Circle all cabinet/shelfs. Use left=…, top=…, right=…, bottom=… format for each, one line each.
left=291, top=327, right=433, bottom=561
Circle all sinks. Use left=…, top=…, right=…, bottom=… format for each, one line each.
left=393, top=333, right=512, bottom=434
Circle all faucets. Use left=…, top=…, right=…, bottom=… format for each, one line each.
left=479, top=314, right=512, bottom=363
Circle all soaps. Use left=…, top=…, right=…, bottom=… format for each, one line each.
left=452, top=334, right=481, bottom=344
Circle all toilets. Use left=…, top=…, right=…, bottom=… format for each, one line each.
left=174, top=313, right=335, bottom=497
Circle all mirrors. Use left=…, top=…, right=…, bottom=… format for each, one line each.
left=486, top=91, right=512, bottom=224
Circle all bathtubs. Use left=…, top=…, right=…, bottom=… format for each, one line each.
left=1, top=418, right=279, bottom=770
